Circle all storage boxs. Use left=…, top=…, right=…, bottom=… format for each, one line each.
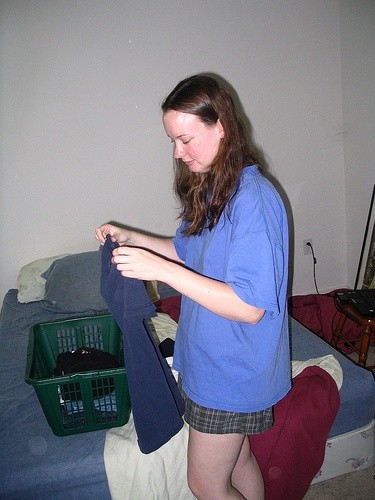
left=24, top=312, right=131, bottom=437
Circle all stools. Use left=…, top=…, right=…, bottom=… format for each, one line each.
left=331, top=290, right=375, bottom=367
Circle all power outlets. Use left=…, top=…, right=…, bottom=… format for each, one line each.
left=302, top=238, right=313, bottom=255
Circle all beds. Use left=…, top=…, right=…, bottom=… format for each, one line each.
left=0, top=290, right=375, bottom=500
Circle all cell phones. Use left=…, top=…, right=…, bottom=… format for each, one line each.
left=336, top=292, right=348, bottom=304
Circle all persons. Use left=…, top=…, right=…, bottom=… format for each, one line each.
left=94, top=74, right=291, bottom=500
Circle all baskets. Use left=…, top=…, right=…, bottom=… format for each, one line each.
left=23, top=313, right=131, bottom=436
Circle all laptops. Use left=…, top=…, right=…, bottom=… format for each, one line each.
left=348, top=289, right=375, bottom=317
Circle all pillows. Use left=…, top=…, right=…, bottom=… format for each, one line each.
left=17, top=254, right=75, bottom=303
left=40, top=251, right=160, bottom=314
